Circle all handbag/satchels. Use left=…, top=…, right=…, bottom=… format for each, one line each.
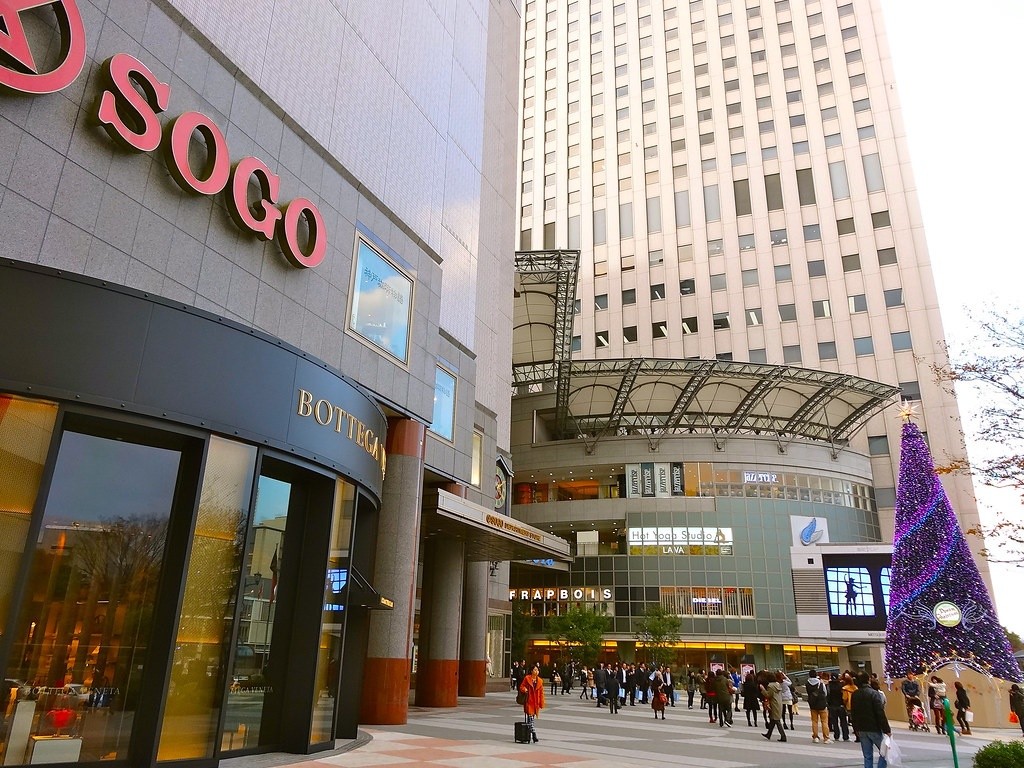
left=879, top=734, right=902, bottom=766
left=659, top=683, right=668, bottom=702
left=1008, top=711, right=1019, bottom=723
left=965, top=709, right=973, bottom=722
left=516, top=691, right=528, bottom=705
left=553, top=674, right=561, bottom=681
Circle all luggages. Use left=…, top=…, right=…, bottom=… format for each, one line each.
left=514, top=691, right=531, bottom=744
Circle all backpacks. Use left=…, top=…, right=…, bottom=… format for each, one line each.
left=806, top=682, right=826, bottom=710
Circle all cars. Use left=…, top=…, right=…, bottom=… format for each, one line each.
left=63, top=683, right=105, bottom=709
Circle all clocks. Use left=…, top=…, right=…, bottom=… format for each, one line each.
left=494, top=466, right=507, bottom=508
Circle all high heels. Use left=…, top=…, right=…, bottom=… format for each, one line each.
left=533, top=733, right=538, bottom=743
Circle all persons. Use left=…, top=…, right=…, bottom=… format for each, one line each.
left=1010, top=685, right=1024, bottom=736
left=511, top=659, right=885, bottom=742
left=521, top=665, right=545, bottom=742
left=928, top=677, right=962, bottom=737
left=628, top=663, right=637, bottom=706
left=806, top=670, right=834, bottom=744
left=685, top=670, right=697, bottom=709
left=714, top=669, right=733, bottom=728
left=594, top=664, right=608, bottom=707
left=792, top=688, right=800, bottom=715
left=778, top=670, right=794, bottom=730
left=851, top=670, right=892, bottom=768
left=740, top=674, right=760, bottom=727
left=928, top=678, right=946, bottom=700
left=580, top=665, right=588, bottom=700
left=760, top=674, right=787, bottom=742
left=650, top=672, right=667, bottom=719
left=901, top=671, right=927, bottom=727
left=954, top=682, right=971, bottom=734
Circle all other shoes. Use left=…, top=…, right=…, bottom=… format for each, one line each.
left=935, top=726, right=946, bottom=734
left=761, top=731, right=772, bottom=740
left=621, top=704, right=627, bottom=706
left=655, top=716, right=658, bottom=719
left=586, top=698, right=588, bottom=700
left=746, top=711, right=752, bottom=726
left=783, top=723, right=788, bottom=729
left=671, top=704, right=676, bottom=707
left=566, top=692, right=570, bottom=695
left=735, top=707, right=740, bottom=711
left=689, top=705, right=693, bottom=709
left=961, top=729, right=971, bottom=734
left=724, top=721, right=732, bottom=727
left=776, top=735, right=786, bottom=742
left=824, top=739, right=834, bottom=743
left=662, top=717, right=665, bottom=720
left=835, top=739, right=839, bottom=741
left=580, top=696, right=582, bottom=699
left=700, top=706, right=707, bottom=709
left=765, top=722, right=772, bottom=729
left=792, top=712, right=794, bottom=714
left=796, top=712, right=799, bottom=715
left=590, top=696, right=592, bottom=699
left=561, top=692, right=564, bottom=695
left=753, top=712, right=757, bottom=727
left=845, top=739, right=852, bottom=742
left=791, top=724, right=794, bottom=730
left=596, top=705, right=601, bottom=707
left=710, top=718, right=716, bottom=723
left=813, top=738, right=819, bottom=743
left=630, top=703, right=636, bottom=706
